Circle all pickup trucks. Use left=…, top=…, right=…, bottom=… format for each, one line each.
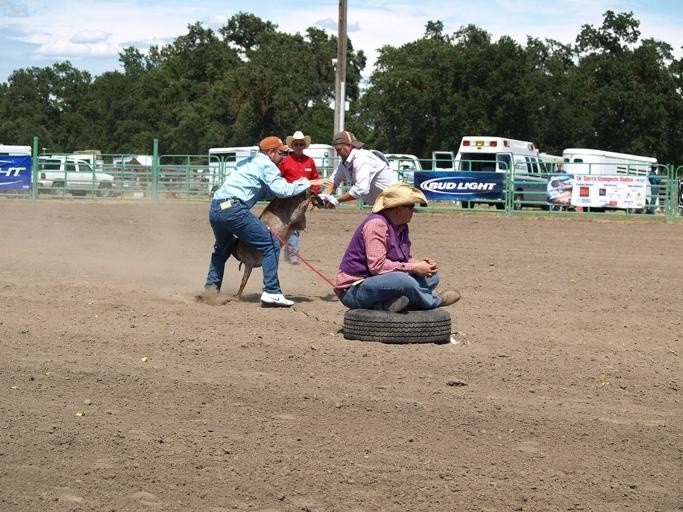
left=29, top=156, right=114, bottom=197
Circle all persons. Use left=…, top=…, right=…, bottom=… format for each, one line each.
left=277, top=128, right=321, bottom=266
left=647, top=163, right=662, bottom=214
left=332, top=182, right=461, bottom=314
left=202, top=135, right=333, bottom=308
left=319, top=131, right=398, bottom=209
left=555, top=163, right=567, bottom=173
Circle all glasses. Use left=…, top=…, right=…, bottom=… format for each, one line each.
left=294, top=143, right=304, bottom=146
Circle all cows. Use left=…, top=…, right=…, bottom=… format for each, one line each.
left=213, top=188, right=335, bottom=299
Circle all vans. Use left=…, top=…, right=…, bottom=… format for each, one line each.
left=431, top=136, right=552, bottom=211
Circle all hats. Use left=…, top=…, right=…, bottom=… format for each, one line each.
left=286, top=131, right=311, bottom=148
left=259, top=136, right=288, bottom=152
left=372, top=183, right=427, bottom=213
left=334, top=131, right=364, bottom=148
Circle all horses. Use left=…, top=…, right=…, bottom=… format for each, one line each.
left=127, top=158, right=179, bottom=199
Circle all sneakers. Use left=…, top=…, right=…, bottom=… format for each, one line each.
left=439, top=289, right=460, bottom=306
left=290, top=254, right=299, bottom=264
left=260, top=292, right=294, bottom=306
